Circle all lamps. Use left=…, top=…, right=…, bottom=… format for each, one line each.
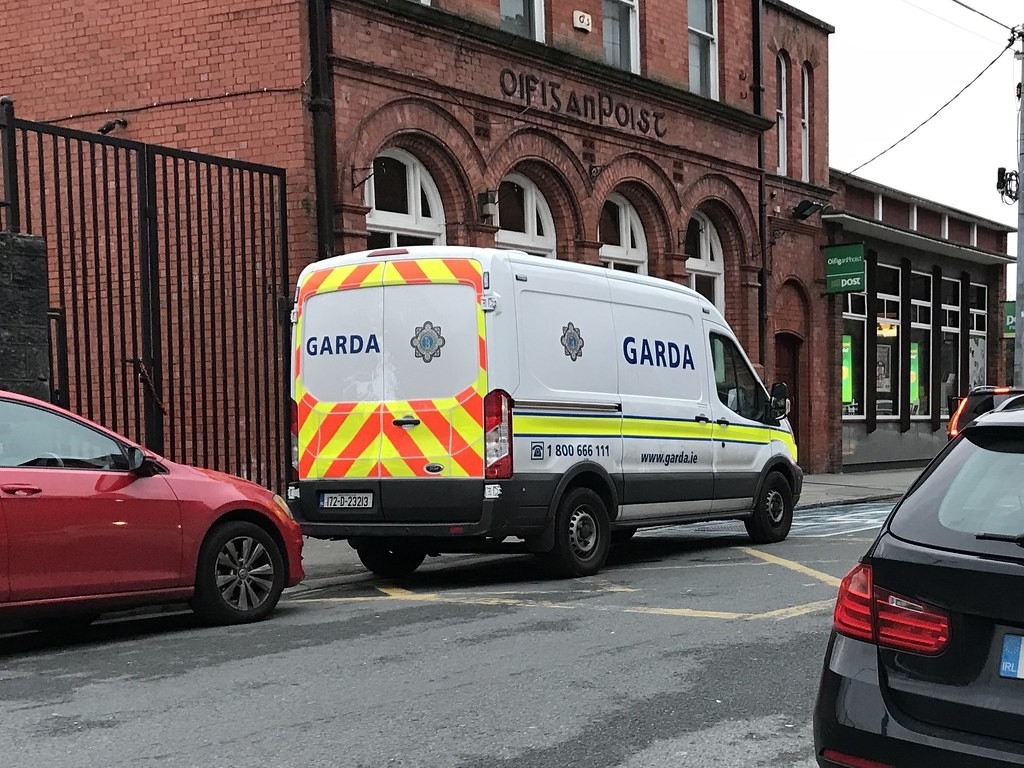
left=791, top=198, right=823, bottom=220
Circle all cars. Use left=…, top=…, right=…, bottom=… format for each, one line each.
left=0, top=389, right=305, bottom=623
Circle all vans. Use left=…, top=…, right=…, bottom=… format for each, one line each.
left=289, top=246, right=802, bottom=575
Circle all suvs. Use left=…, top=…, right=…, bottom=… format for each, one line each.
left=812, top=392, right=1024, bottom=768
left=948, top=387, right=1024, bottom=440
